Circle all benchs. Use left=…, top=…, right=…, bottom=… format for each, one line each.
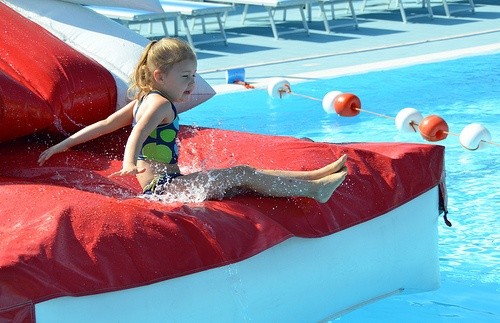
left=82, top=1, right=476, bottom=53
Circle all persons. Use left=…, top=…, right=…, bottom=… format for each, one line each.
left=37, top=38, right=348, bottom=203
